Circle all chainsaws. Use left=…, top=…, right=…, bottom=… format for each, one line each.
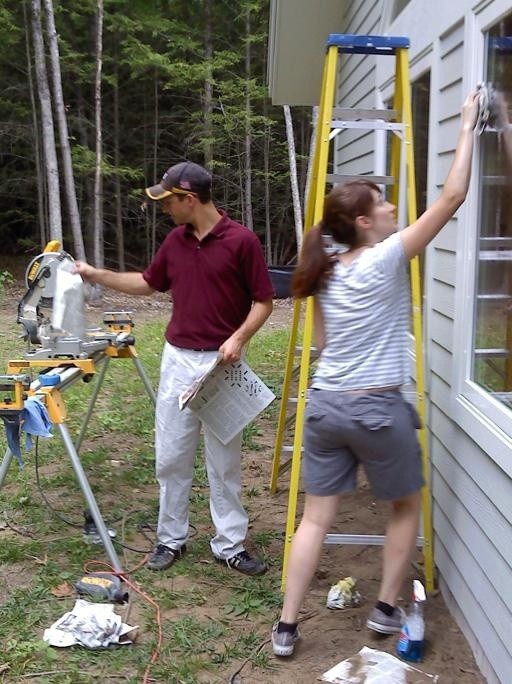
left=17, top=239, right=135, bottom=360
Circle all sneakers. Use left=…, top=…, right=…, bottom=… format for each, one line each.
left=215, top=550, right=267, bottom=576
left=271, top=622, right=300, bottom=656
left=147, top=543, right=186, bottom=571
left=367, top=604, right=407, bottom=634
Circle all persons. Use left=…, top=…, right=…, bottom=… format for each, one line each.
left=66, top=158, right=274, bottom=578
left=268, top=87, right=486, bottom=659
left=489, top=89, right=512, bottom=164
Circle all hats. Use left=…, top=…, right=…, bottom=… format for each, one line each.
left=146, top=162, right=213, bottom=199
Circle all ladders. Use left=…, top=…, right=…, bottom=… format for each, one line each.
left=269, top=34, right=435, bottom=592
